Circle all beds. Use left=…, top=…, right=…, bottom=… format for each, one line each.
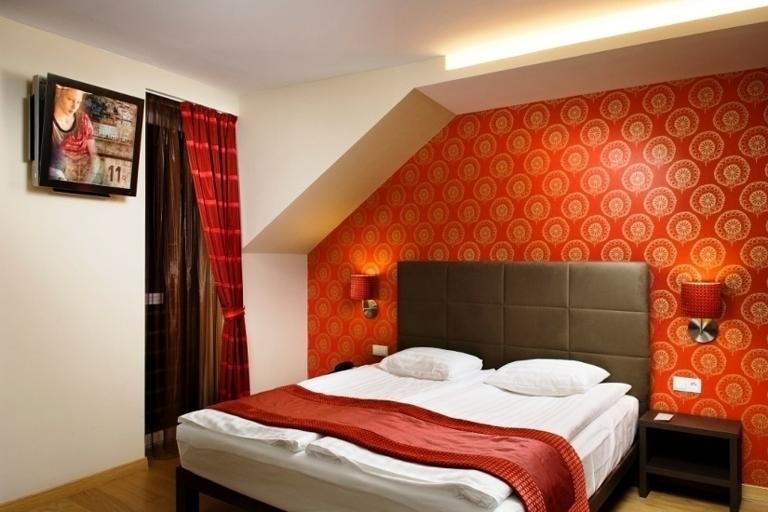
left=176, top=261, right=651, bottom=511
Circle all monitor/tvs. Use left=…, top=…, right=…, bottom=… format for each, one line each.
left=29, top=73, right=144, bottom=197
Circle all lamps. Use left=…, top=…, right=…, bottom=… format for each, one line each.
left=350, top=274, right=379, bottom=319
left=680, top=281, right=723, bottom=343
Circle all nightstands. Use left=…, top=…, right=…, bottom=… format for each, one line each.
left=639, top=408, right=742, bottom=512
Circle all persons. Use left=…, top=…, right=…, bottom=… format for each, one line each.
left=49, top=83, right=100, bottom=185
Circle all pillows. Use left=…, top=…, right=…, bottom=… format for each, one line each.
left=378, top=347, right=484, bottom=381
left=482, top=358, right=611, bottom=397
